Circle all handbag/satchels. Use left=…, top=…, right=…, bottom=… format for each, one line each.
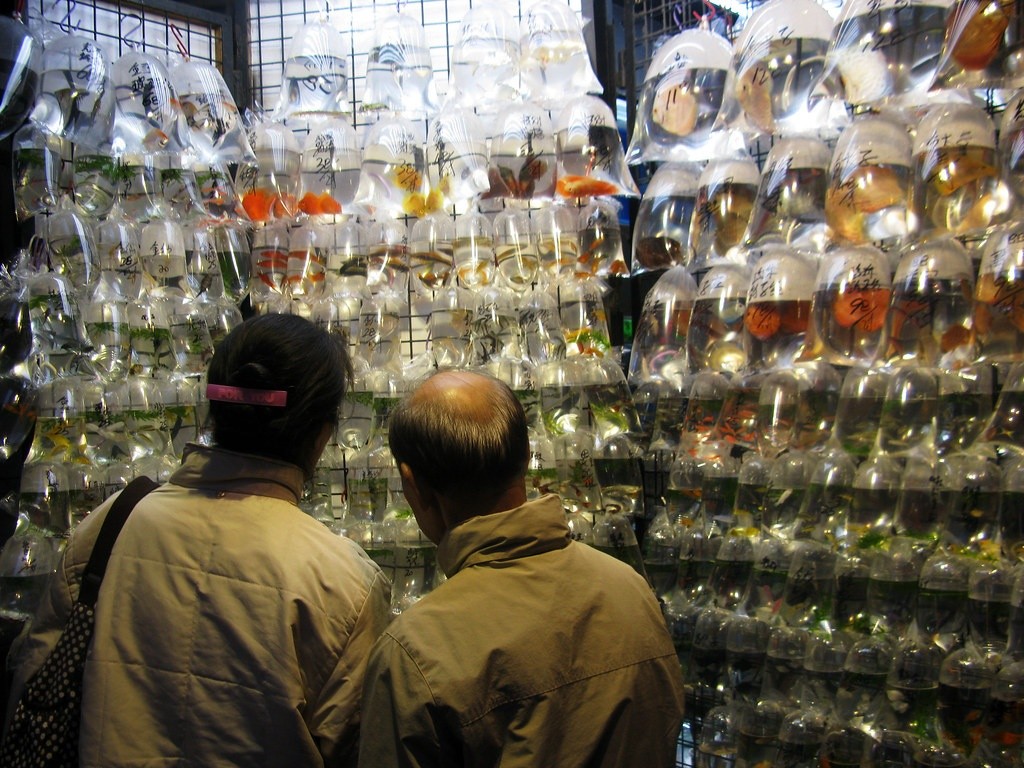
left=1, top=474, right=162, bottom=767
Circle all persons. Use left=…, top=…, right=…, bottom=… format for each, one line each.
left=1, top=311, right=394, bottom=768
left=357, top=367, right=684, bottom=766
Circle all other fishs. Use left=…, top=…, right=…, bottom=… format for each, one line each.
left=0, top=1, right=1024, bottom=768
